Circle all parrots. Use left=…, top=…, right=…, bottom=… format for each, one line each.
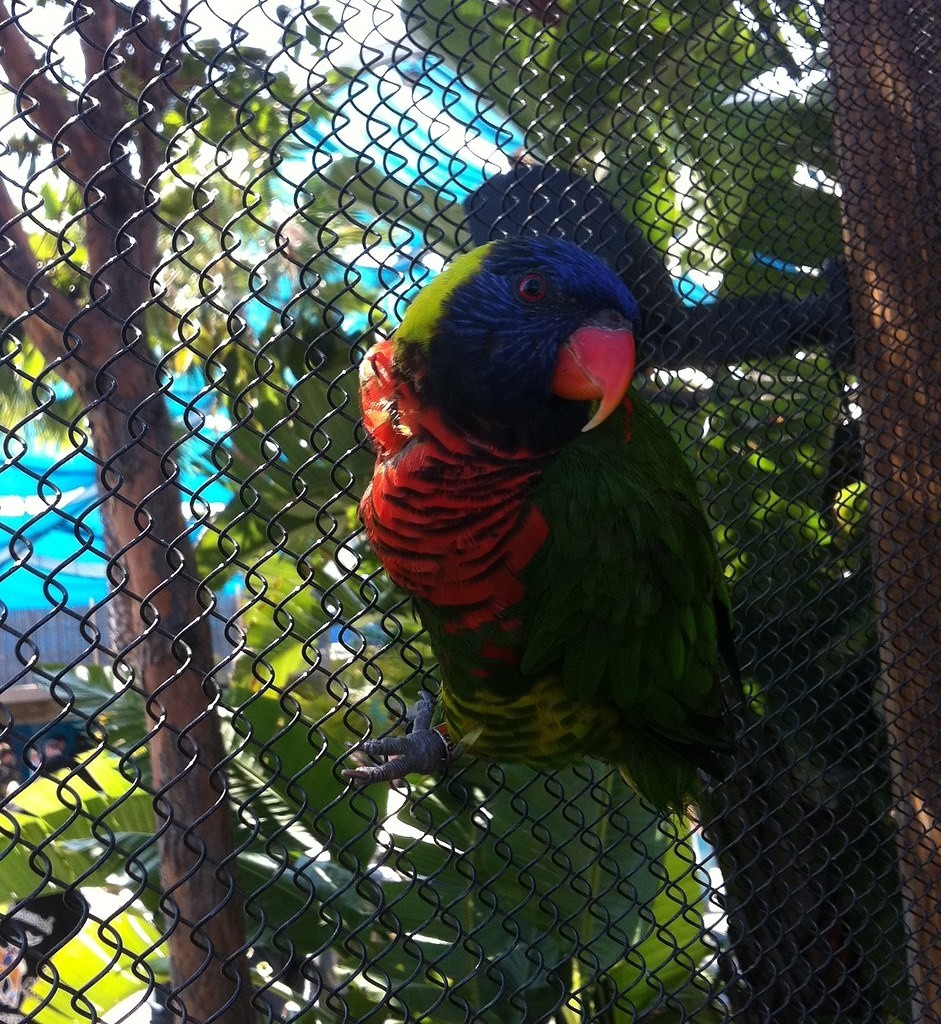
left=341, top=236, right=748, bottom=828
